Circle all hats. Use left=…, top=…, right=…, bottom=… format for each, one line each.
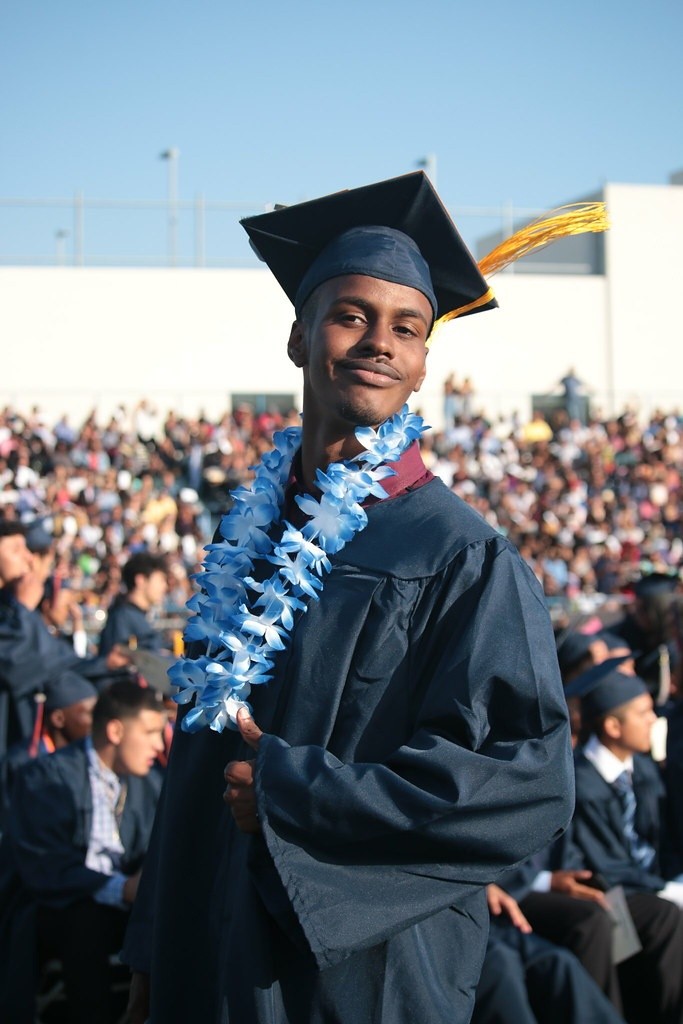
left=18, top=658, right=99, bottom=757
left=554, top=573, right=680, bottom=671
left=22, top=513, right=55, bottom=554
left=566, top=655, right=653, bottom=723
left=237, top=169, right=610, bottom=338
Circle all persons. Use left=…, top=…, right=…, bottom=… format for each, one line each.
left=0, top=369, right=683, bottom=1024
left=131, top=169, right=574, bottom=1024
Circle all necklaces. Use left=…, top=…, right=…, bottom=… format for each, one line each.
left=168, top=401, right=433, bottom=736
